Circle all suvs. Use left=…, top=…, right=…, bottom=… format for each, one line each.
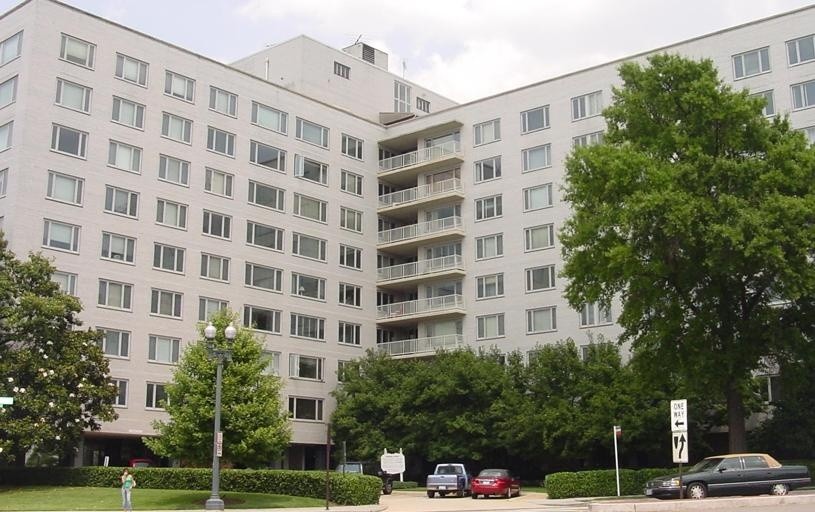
left=335, top=460, right=394, bottom=497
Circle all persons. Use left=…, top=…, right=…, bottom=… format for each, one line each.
left=121, top=468, right=136, bottom=512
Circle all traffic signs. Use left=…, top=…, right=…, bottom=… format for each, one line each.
left=670, top=399, right=690, bottom=432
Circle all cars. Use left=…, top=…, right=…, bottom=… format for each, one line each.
left=468, top=466, right=522, bottom=499
left=126, top=456, right=157, bottom=468
left=643, top=450, right=811, bottom=499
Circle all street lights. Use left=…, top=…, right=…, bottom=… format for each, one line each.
left=201, top=319, right=237, bottom=510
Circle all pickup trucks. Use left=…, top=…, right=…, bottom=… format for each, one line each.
left=424, top=460, right=473, bottom=500
